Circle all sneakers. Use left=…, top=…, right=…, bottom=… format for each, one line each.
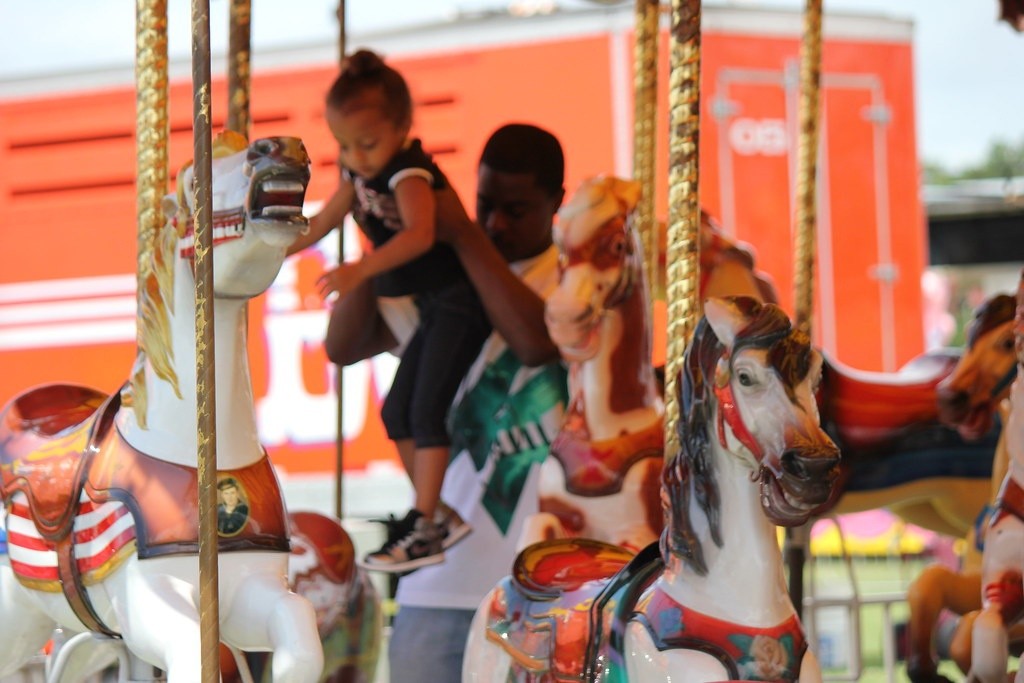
left=365, top=508, right=446, bottom=572
left=432, top=502, right=470, bottom=549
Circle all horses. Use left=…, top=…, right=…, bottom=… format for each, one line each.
left=456, top=172, right=1024, bottom=683
left=1, top=127, right=384, bottom=683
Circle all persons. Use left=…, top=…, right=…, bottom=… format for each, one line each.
left=285, top=48, right=494, bottom=575
left=326, top=123, right=568, bottom=683
